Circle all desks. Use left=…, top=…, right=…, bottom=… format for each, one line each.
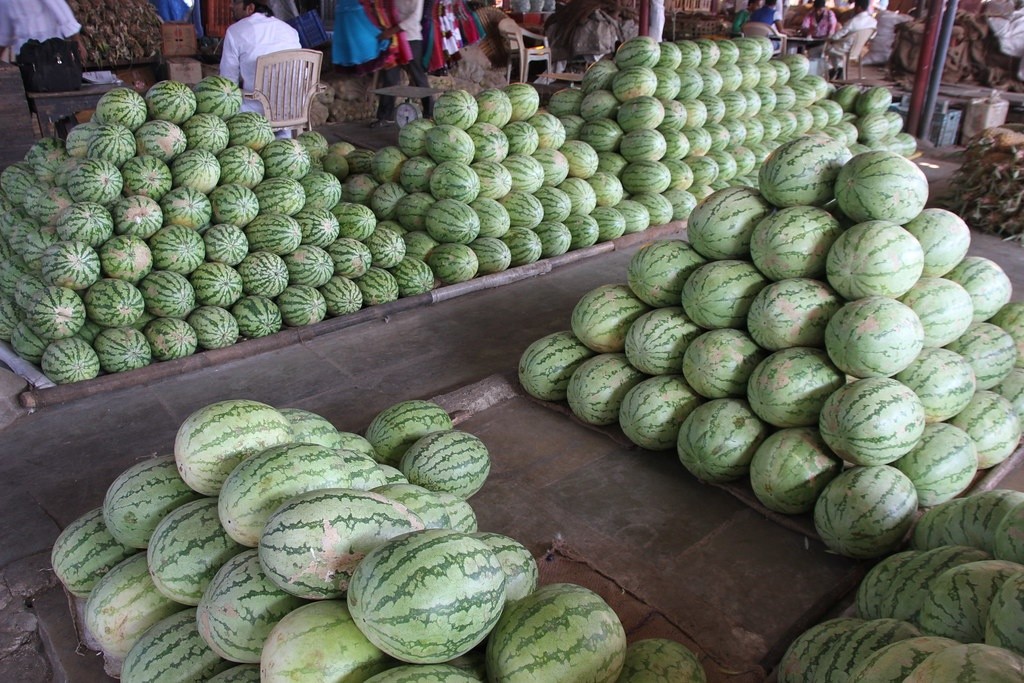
left=23, top=81, right=134, bottom=139
left=785, top=34, right=826, bottom=55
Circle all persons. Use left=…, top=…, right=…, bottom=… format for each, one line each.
left=218, top=0, right=303, bottom=140
left=807, top=0, right=879, bottom=81
left=750, top=0, right=794, bottom=57
left=735, top=0, right=857, bottom=38
left=731, top=0, right=762, bottom=41
left=369, top=0, right=435, bottom=129
left=1, top=0, right=89, bottom=67
left=797, top=0, right=837, bottom=56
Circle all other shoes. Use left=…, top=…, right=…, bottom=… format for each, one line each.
left=829, top=68, right=836, bottom=80
left=422, top=112, right=434, bottom=119
left=836, top=68, right=843, bottom=80
left=370, top=119, right=395, bottom=128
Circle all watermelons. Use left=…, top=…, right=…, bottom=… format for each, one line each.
left=1, top=35, right=1024, bottom=683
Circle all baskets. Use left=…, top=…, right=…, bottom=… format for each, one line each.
left=666, top=16, right=720, bottom=40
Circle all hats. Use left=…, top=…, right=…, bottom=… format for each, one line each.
left=873, top=0, right=889, bottom=11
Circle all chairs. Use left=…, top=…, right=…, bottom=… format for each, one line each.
left=235, top=49, right=324, bottom=138
left=499, top=17, right=553, bottom=86
left=830, top=26, right=877, bottom=83
left=741, top=22, right=789, bottom=60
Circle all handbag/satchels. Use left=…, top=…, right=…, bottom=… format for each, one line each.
left=16, top=37, right=83, bottom=93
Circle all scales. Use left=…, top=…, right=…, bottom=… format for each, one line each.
left=536, top=73, right=584, bottom=90
left=369, top=84, right=446, bottom=130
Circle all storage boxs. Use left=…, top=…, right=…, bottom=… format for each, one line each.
left=891, top=101, right=964, bottom=147
left=164, top=59, right=203, bottom=85
left=160, top=22, right=196, bottom=55
